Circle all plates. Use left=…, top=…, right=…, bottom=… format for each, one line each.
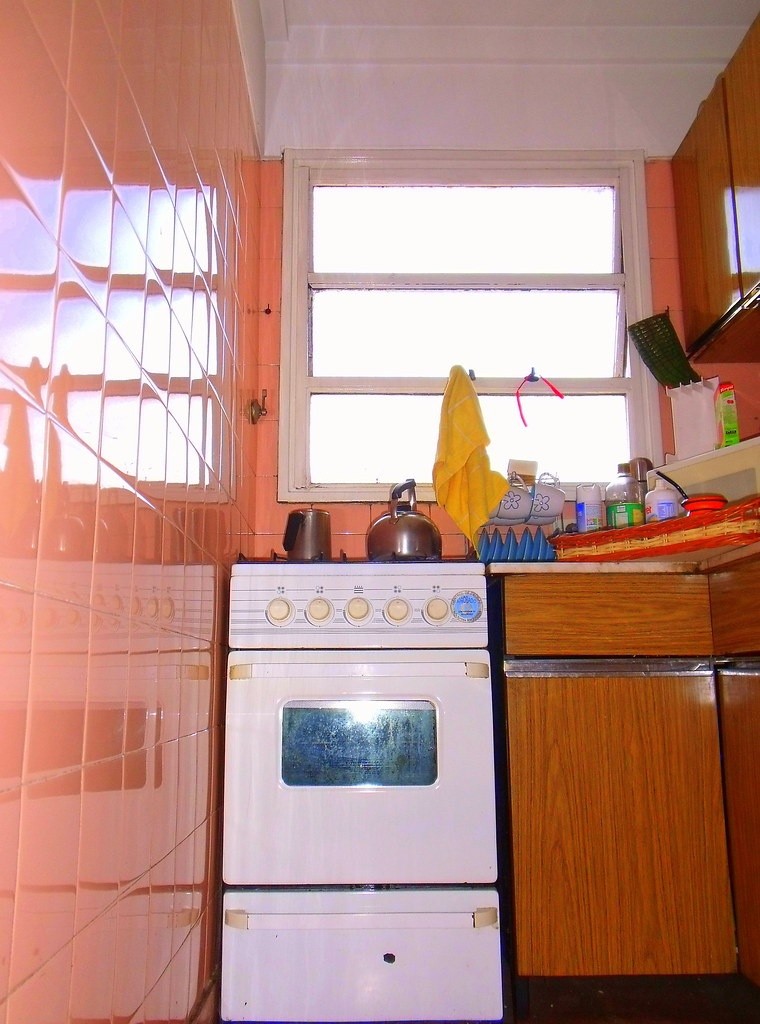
left=503, top=526, right=519, bottom=562
left=533, top=526, right=552, bottom=562
left=517, top=527, right=534, bottom=562
left=476, top=527, right=490, bottom=565
left=488, top=527, right=507, bottom=562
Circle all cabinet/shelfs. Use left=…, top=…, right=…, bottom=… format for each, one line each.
left=488, top=559, right=760, bottom=1024
left=670, top=14, right=760, bottom=367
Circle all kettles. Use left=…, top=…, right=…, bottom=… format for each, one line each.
left=364, top=479, right=444, bottom=561
left=281, top=503, right=330, bottom=563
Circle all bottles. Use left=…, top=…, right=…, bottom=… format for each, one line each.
left=604, top=463, right=645, bottom=530
left=644, top=477, right=679, bottom=526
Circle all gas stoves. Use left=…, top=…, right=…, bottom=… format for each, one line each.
left=227, top=550, right=489, bottom=650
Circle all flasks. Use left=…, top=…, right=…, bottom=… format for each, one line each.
left=626, top=458, right=656, bottom=502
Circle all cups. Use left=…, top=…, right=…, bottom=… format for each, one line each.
left=479, top=473, right=565, bottom=526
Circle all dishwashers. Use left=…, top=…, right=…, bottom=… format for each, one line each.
left=223, top=646, right=499, bottom=891
